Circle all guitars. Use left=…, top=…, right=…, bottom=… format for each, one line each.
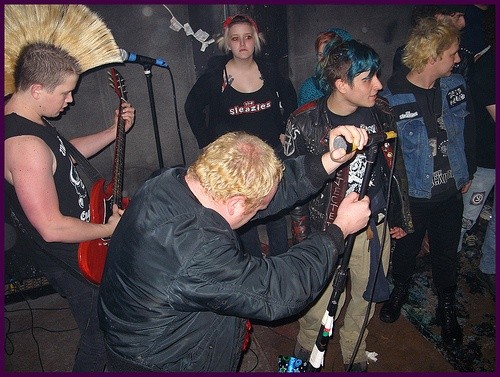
left=78, top=67, right=130, bottom=286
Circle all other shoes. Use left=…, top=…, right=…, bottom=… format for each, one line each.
left=463, top=231, right=483, bottom=258
left=294, top=341, right=311, bottom=360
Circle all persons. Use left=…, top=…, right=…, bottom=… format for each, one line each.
left=280, top=37, right=414, bottom=373
left=3, top=42, right=135, bottom=372
left=393, top=5, right=477, bottom=81
left=456, top=44, right=498, bottom=284
left=463, top=4, right=496, bottom=60
left=184, top=15, right=298, bottom=257
left=98, top=131, right=371, bottom=372
left=379, top=18, right=477, bottom=345
left=298, top=27, right=352, bottom=107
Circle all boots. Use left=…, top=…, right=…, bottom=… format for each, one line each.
left=380, top=274, right=411, bottom=322
left=437, top=294, right=464, bottom=343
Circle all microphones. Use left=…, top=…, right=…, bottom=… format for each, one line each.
left=119, top=49, right=170, bottom=69
left=332, top=131, right=399, bottom=155
